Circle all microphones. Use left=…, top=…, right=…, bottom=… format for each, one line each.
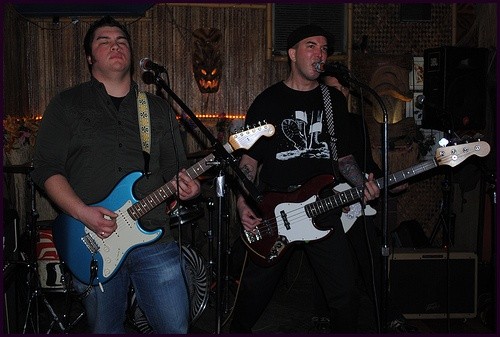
left=139, top=58, right=166, bottom=74
left=316, top=61, right=349, bottom=73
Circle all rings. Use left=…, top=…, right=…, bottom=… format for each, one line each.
left=101, top=232, right=105, bottom=236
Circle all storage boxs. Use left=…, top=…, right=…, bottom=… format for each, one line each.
left=384, top=247, right=478, bottom=320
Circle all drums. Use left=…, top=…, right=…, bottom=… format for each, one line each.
left=125, top=240, right=210, bottom=334
left=23, top=220, right=68, bottom=298
left=166, top=196, right=204, bottom=227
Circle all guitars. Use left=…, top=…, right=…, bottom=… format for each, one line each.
left=52, top=120, right=275, bottom=287
left=238, top=132, right=491, bottom=263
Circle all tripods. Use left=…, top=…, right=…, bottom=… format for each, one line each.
left=22, top=179, right=72, bottom=336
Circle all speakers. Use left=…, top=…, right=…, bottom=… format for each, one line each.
left=422, top=45, right=472, bottom=128
left=387, top=248, right=478, bottom=321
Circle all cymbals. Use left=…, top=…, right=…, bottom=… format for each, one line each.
left=1, top=160, right=35, bottom=174
left=186, top=148, right=216, bottom=157
left=196, top=176, right=214, bottom=181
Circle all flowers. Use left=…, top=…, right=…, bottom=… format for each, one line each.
left=2, top=114, right=43, bottom=164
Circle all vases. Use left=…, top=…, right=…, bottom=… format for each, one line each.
left=6, top=138, right=34, bottom=164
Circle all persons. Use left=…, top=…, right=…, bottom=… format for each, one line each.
left=228, top=24, right=402, bottom=337
left=29, top=19, right=203, bottom=337
